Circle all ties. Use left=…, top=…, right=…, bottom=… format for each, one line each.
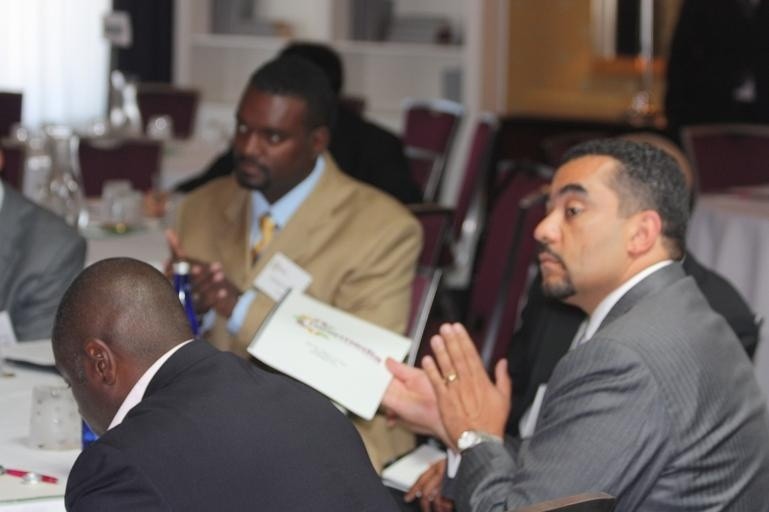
left=253, top=215, right=274, bottom=258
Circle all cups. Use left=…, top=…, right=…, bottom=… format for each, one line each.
left=102, top=179, right=142, bottom=233
left=30, top=387, right=81, bottom=450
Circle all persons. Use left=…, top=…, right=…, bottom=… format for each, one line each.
left=379, top=131, right=758, bottom=453
left=142, top=42, right=422, bottom=219
left=403, top=139, right=769, bottom=512
left=2, top=131, right=87, bottom=343
left=161, top=60, right=428, bottom=482
left=52, top=258, right=402, bottom=511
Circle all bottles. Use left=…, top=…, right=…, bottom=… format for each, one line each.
left=81, top=419, right=101, bottom=454
left=629, top=56, right=656, bottom=121
left=172, top=261, right=197, bottom=338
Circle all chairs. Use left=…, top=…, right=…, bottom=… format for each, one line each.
left=678, top=123, right=768, bottom=194
left=77, top=137, right=161, bottom=199
left=505, top=491, right=620, bottom=510
left=0, top=92, right=26, bottom=185
left=136, top=85, right=200, bottom=140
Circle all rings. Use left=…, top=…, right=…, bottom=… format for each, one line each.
left=443, top=374, right=457, bottom=384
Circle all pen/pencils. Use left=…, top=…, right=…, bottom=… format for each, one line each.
left=0, top=469, right=58, bottom=483
